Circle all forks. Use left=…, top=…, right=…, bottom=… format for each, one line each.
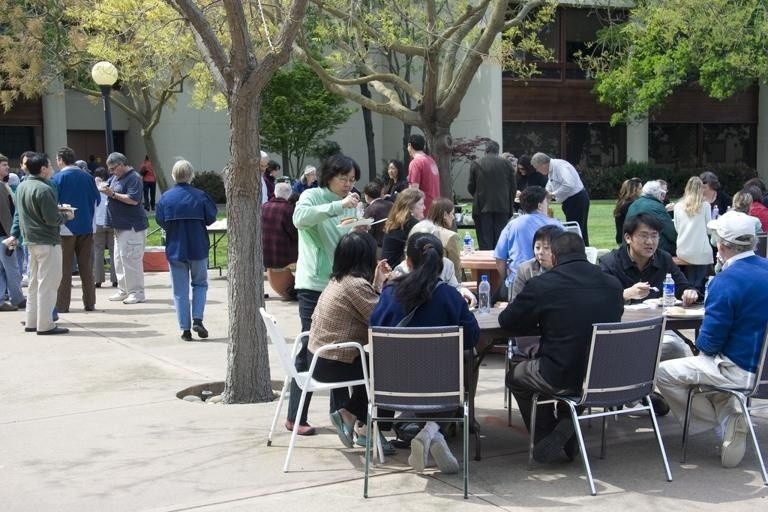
left=646, top=285, right=660, bottom=293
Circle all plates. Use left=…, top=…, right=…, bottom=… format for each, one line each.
left=662, top=310, right=705, bottom=319
left=335, top=218, right=373, bottom=228
left=58, top=206, right=78, bottom=212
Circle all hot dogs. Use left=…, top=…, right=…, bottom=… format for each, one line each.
left=340, top=217, right=358, bottom=226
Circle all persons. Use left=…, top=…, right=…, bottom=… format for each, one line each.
left=0, top=146, right=157, bottom=335
left=153, top=156, right=216, bottom=337
left=258, top=133, right=768, bottom=467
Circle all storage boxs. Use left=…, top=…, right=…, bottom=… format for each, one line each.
left=140, top=244, right=170, bottom=274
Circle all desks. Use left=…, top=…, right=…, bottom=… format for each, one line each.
left=204, top=217, right=228, bottom=278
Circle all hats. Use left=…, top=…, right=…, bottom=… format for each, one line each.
left=707, top=211, right=755, bottom=245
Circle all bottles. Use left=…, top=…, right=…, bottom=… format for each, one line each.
left=704, top=276, right=714, bottom=299
left=478, top=275, right=490, bottom=314
left=712, top=205, right=718, bottom=218
left=662, top=273, right=675, bottom=310
left=463, top=232, right=472, bottom=256
left=726, top=206, right=730, bottom=212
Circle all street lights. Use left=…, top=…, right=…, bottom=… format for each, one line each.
left=90, top=60, right=122, bottom=182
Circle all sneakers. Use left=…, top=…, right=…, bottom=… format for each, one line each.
left=534, top=393, right=749, bottom=468
left=286, top=411, right=461, bottom=474
left=0, top=292, right=207, bottom=341
